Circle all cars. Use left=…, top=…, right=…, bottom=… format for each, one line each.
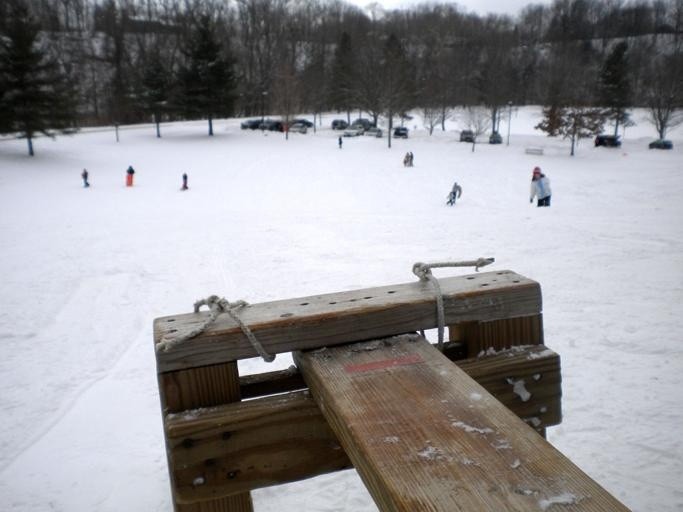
left=460, top=130, right=475, bottom=143
left=241, top=118, right=314, bottom=134
left=331, top=117, right=382, bottom=138
left=648, top=139, right=672, bottom=150
left=488, top=133, right=502, bottom=144
left=393, top=127, right=408, bottom=139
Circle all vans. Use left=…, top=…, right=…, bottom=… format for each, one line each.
left=595, top=134, right=621, bottom=148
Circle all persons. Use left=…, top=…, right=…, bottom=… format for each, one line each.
left=81, top=168, right=90, bottom=188
left=181, top=172, right=188, bottom=189
left=403, top=151, right=414, bottom=168
left=126, top=164, right=135, bottom=186
left=530, top=166, right=551, bottom=206
left=446, top=182, right=462, bottom=206
left=339, top=136, right=342, bottom=148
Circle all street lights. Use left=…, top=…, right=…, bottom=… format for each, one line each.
left=260, top=89, right=268, bottom=125
left=505, top=101, right=512, bottom=146
